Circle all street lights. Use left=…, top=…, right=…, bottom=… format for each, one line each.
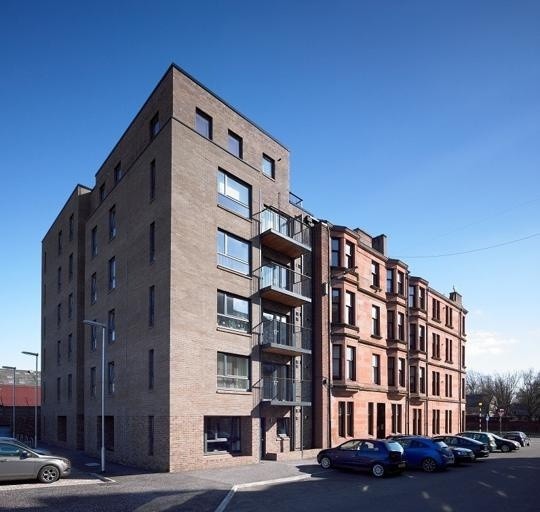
left=479, top=402, right=483, bottom=432
left=22, top=351, right=38, bottom=449
left=3, top=366, right=16, bottom=438
left=83, top=319, right=108, bottom=473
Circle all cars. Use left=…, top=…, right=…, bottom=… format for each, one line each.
left=383, top=436, right=455, bottom=473
left=0, top=437, right=52, bottom=456
left=431, top=434, right=489, bottom=463
left=503, top=431, right=531, bottom=447
left=455, top=432, right=497, bottom=453
left=317, top=437, right=408, bottom=478
left=387, top=433, right=475, bottom=467
left=0, top=442, right=72, bottom=484
left=464, top=430, right=521, bottom=453
